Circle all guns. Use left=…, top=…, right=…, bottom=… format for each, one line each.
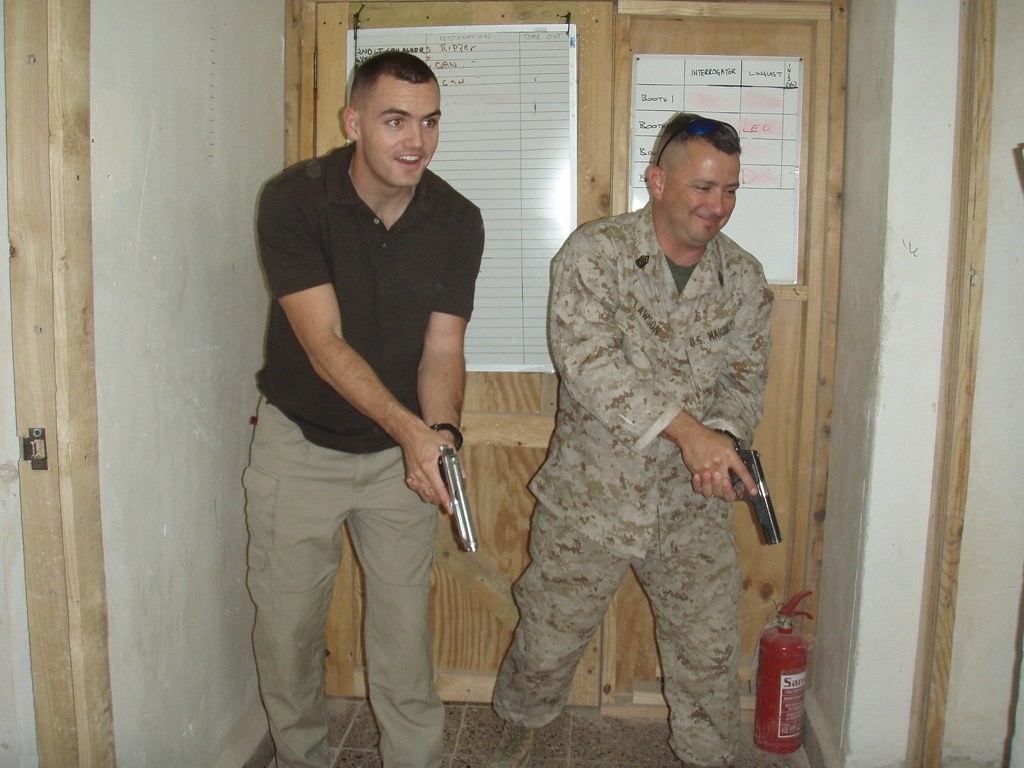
left=729, top=439, right=783, bottom=546
left=437, top=442, right=479, bottom=553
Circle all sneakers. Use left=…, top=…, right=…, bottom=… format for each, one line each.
left=484, top=721, right=533, bottom=768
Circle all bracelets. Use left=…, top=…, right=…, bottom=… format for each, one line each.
left=430, top=423, right=464, bottom=452
left=715, top=427, right=742, bottom=449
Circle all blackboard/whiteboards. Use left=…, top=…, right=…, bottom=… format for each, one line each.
left=623, top=51, right=805, bottom=291
left=343, top=22, right=580, bottom=375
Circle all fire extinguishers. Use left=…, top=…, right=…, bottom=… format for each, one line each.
left=753, top=588, right=815, bottom=756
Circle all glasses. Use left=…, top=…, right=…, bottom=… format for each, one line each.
left=657, top=118, right=738, bottom=166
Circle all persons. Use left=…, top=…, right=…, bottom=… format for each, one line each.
left=486, top=113, right=774, bottom=768
left=240, top=52, right=485, bottom=768
